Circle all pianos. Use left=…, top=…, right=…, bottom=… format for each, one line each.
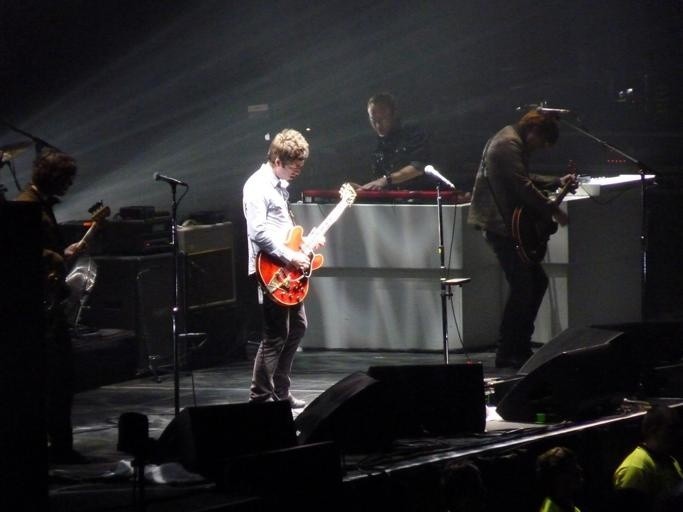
left=302, top=185, right=473, bottom=205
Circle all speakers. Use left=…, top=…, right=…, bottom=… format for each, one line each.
left=367, top=363, right=485, bottom=439
left=497, top=325, right=624, bottom=422
left=157, top=399, right=296, bottom=492
left=295, top=370, right=380, bottom=450
left=602, top=320, right=683, bottom=398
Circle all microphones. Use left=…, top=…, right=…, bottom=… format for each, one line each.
left=424, top=165, right=456, bottom=191
left=154, top=172, right=183, bottom=186
left=536, top=106, right=571, bottom=115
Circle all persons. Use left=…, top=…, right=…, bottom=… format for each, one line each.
left=535, top=446, right=591, bottom=512
left=353, top=93, right=435, bottom=192
left=466, top=106, right=582, bottom=367
left=612, top=404, right=682, bottom=511
left=242, top=126, right=327, bottom=412
left=0, top=144, right=93, bottom=465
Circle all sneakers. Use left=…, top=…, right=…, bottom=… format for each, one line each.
left=280, top=393, right=306, bottom=409
left=494, top=347, right=534, bottom=369
left=51, top=446, right=84, bottom=464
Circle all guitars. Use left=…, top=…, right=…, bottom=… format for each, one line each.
left=45, top=203, right=110, bottom=340
left=511, top=174, right=579, bottom=264
left=256, top=184, right=355, bottom=306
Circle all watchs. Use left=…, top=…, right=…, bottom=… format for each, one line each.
left=382, top=172, right=393, bottom=186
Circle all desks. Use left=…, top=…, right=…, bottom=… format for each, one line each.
left=290, top=201, right=501, bottom=355
left=529, top=174, right=660, bottom=346
